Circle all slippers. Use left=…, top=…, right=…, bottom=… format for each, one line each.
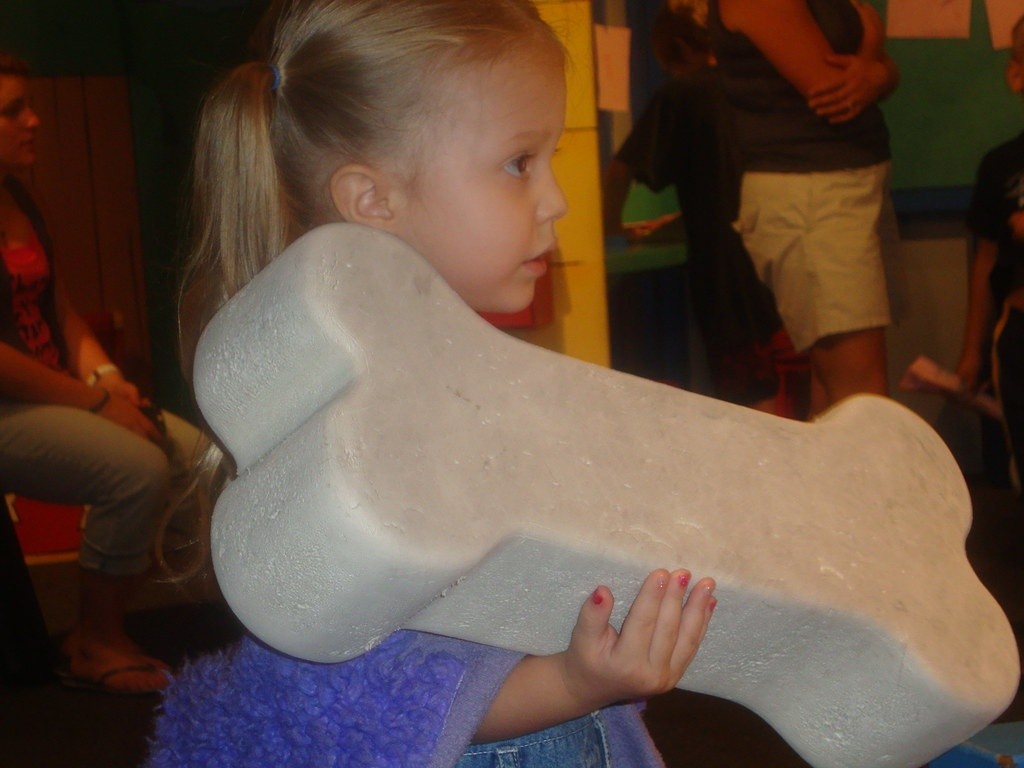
left=60, top=659, right=174, bottom=696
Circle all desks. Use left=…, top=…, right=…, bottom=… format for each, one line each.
left=601, top=223, right=714, bottom=393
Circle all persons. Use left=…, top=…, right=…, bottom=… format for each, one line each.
left=628, top=0, right=901, bottom=423
left=0, top=44, right=231, bottom=693
left=139, top=0, right=719, bottom=768
left=953, top=13, right=1024, bottom=502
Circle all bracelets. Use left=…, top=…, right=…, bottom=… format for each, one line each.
left=90, top=384, right=110, bottom=413
left=86, top=364, right=119, bottom=386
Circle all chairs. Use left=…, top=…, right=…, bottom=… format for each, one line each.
left=721, top=325, right=812, bottom=419
